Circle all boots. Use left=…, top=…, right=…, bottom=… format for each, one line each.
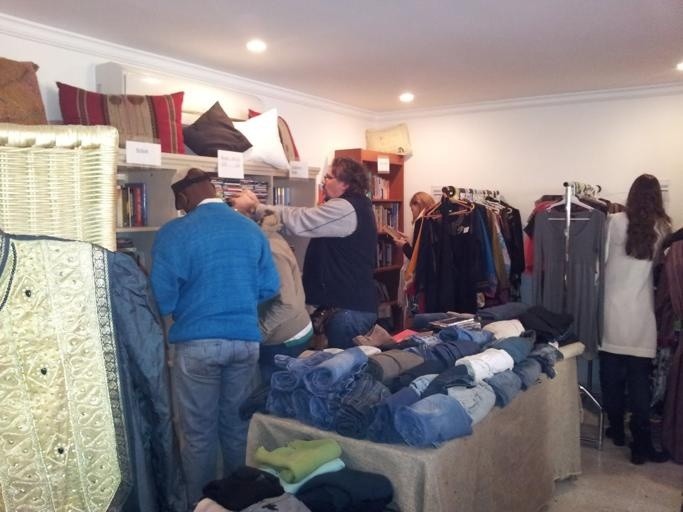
left=629, top=420, right=670, bottom=464
left=606, top=414, right=625, bottom=445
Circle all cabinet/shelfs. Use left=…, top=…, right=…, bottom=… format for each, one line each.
left=117, top=149, right=404, bottom=350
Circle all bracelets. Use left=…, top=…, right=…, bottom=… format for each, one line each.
left=247, top=200, right=259, bottom=216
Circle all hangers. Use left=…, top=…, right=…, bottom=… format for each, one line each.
left=546, top=181, right=607, bottom=221
left=423, top=185, right=512, bottom=219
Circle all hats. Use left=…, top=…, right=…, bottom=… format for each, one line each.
left=170, top=168, right=210, bottom=210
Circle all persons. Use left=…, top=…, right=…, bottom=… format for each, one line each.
left=392, top=192, right=435, bottom=327
left=597, top=172, right=674, bottom=464
left=224, top=191, right=317, bottom=367
left=148, top=165, right=282, bottom=512
left=240, top=157, right=379, bottom=347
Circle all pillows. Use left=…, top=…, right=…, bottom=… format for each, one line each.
left=0, top=57, right=298, bottom=172
left=365, top=124, right=413, bottom=155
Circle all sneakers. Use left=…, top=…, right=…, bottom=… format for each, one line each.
left=649, top=406, right=664, bottom=420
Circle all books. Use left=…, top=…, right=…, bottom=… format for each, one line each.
left=116, top=182, right=148, bottom=252
left=365, top=168, right=402, bottom=304
left=209, top=177, right=293, bottom=207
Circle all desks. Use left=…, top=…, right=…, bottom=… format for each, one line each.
left=246, top=357, right=583, bottom=512
